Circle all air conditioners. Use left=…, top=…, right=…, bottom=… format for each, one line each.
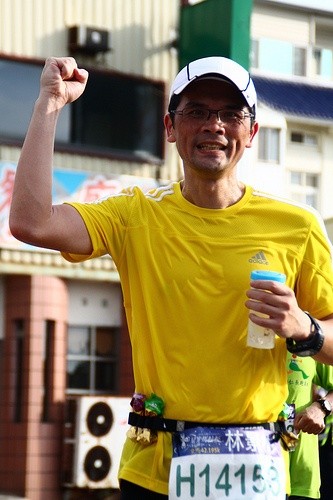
left=59, top=395, right=135, bottom=491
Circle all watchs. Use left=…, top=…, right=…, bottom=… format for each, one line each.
left=314, top=398, right=331, bottom=418
left=285, top=311, right=325, bottom=357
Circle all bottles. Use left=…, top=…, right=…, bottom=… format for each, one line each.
left=248, top=269, right=287, bottom=349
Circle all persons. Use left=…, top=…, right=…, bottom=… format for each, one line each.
left=286, top=352, right=333, bottom=500
left=10, top=55, right=332, bottom=500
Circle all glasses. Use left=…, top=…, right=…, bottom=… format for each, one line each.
left=170, top=104, right=254, bottom=123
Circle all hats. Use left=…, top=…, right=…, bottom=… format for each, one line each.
left=168, top=55, right=259, bottom=120
